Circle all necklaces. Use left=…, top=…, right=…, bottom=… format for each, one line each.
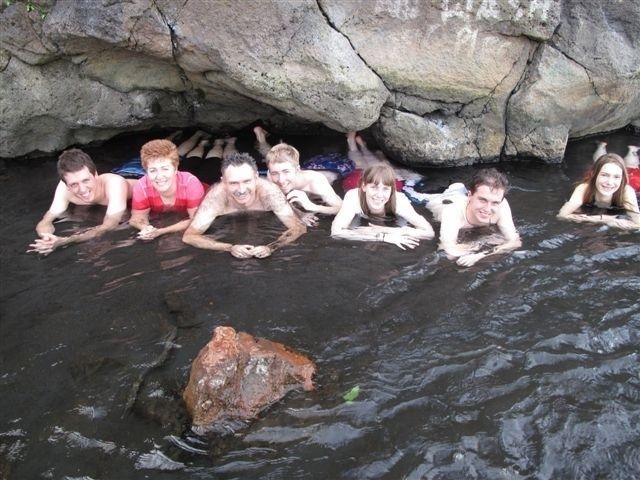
left=465, top=203, right=491, bottom=226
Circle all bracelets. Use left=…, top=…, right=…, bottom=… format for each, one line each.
left=600, top=213, right=602, bottom=220
left=481, top=245, right=493, bottom=259
left=377, top=231, right=385, bottom=243
left=266, top=241, right=282, bottom=255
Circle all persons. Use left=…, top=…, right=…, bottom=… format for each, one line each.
left=127, top=138, right=226, bottom=242
left=555, top=139, right=640, bottom=233
left=330, top=132, right=436, bottom=251
left=181, top=127, right=308, bottom=260
left=266, top=126, right=343, bottom=228
left=24, top=129, right=212, bottom=257
left=402, top=165, right=522, bottom=268
